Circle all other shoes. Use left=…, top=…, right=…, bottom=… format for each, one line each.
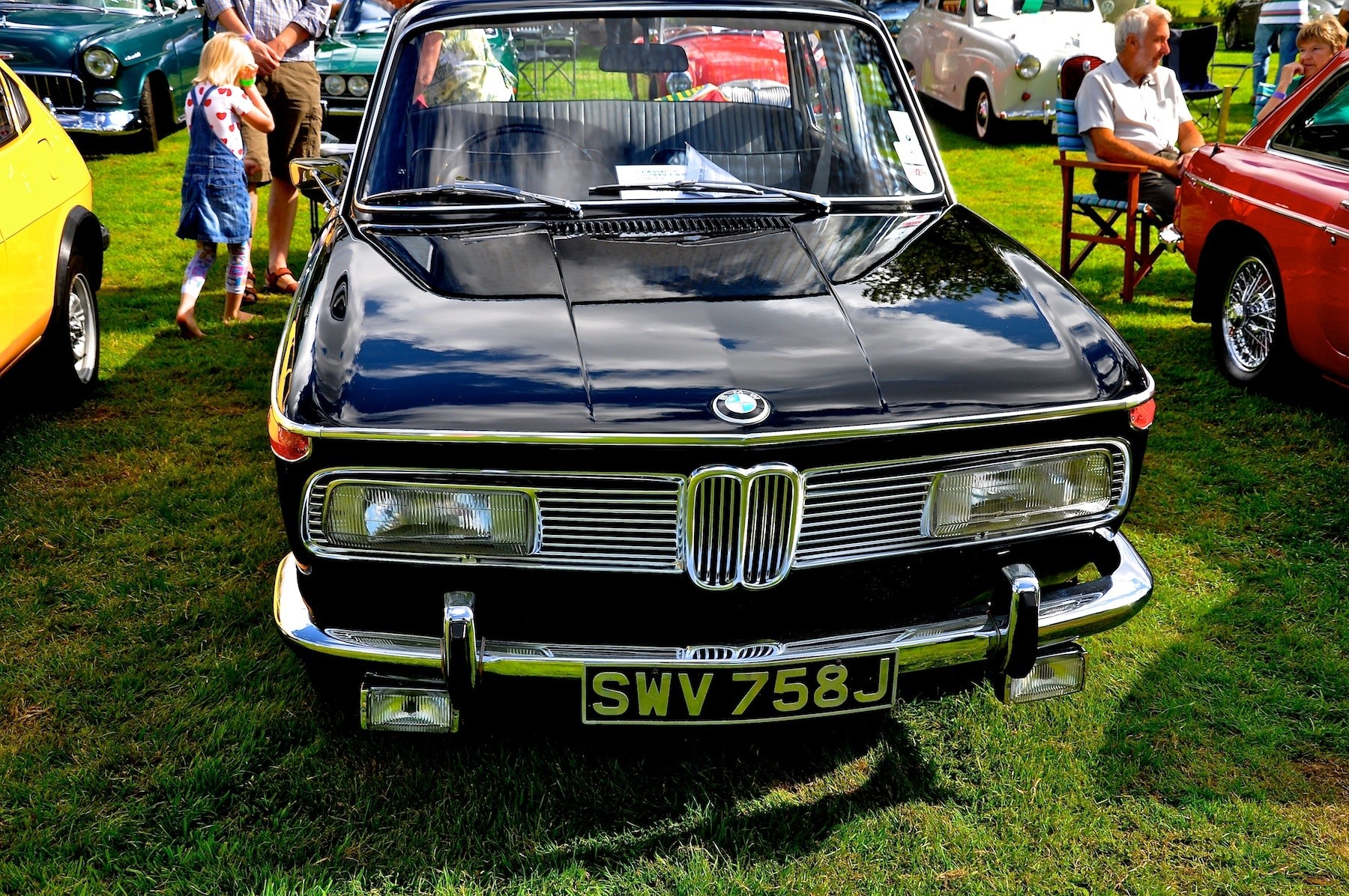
left=1250, top=96, right=1255, bottom=105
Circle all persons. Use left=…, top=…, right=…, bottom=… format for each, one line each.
left=205, top=0, right=518, bottom=306
left=177, top=31, right=265, bottom=338
left=1251, top=12, right=1348, bottom=127
left=1247, top=0, right=1349, bottom=108
left=1074, top=4, right=1206, bottom=226
left=605, top=17, right=632, bottom=46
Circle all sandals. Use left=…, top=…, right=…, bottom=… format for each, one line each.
left=239, top=273, right=258, bottom=306
left=261, top=263, right=299, bottom=296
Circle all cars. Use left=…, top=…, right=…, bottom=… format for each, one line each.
left=633, top=25, right=845, bottom=135
left=1221, top=0, right=1344, bottom=49
left=862, top=0, right=1128, bottom=141
left=314, top=0, right=521, bottom=143
left=263, top=0, right=1162, bottom=739
left=0, top=57, right=111, bottom=399
left=1164, top=44, right=1348, bottom=406
left=0, top=0, right=217, bottom=154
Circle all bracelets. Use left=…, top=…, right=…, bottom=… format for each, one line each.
left=240, top=76, right=256, bottom=86
left=1271, top=91, right=1286, bottom=100
left=1191, top=147, right=1199, bottom=152
left=242, top=33, right=253, bottom=42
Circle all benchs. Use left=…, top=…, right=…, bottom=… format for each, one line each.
left=413, top=98, right=812, bottom=196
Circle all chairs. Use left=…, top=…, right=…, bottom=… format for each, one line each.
left=513, top=26, right=578, bottom=96
left=649, top=147, right=846, bottom=192
left=409, top=147, right=606, bottom=191
left=1253, top=81, right=1276, bottom=124
left=1054, top=98, right=1166, bottom=304
left=1160, top=24, right=1262, bottom=134
